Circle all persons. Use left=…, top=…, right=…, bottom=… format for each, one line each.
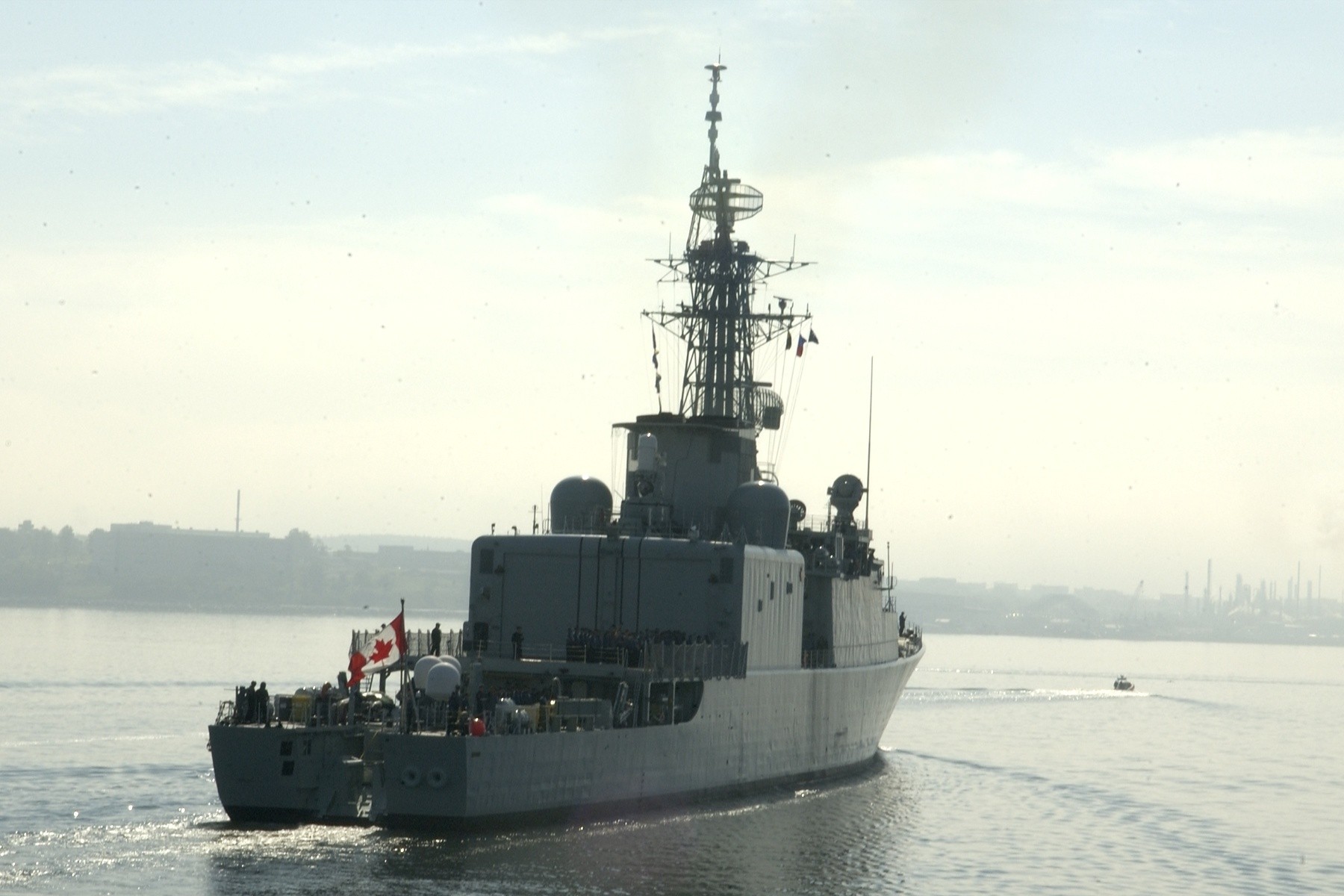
left=899, top=611, right=907, bottom=637
left=403, top=683, right=544, bottom=736
left=380, top=622, right=713, bottom=670
left=237, top=681, right=272, bottom=724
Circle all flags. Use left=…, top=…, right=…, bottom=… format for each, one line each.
left=346, top=611, right=409, bottom=688
left=786, top=328, right=819, bottom=357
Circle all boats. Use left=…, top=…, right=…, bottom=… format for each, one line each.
left=204, top=53, right=924, bottom=837
